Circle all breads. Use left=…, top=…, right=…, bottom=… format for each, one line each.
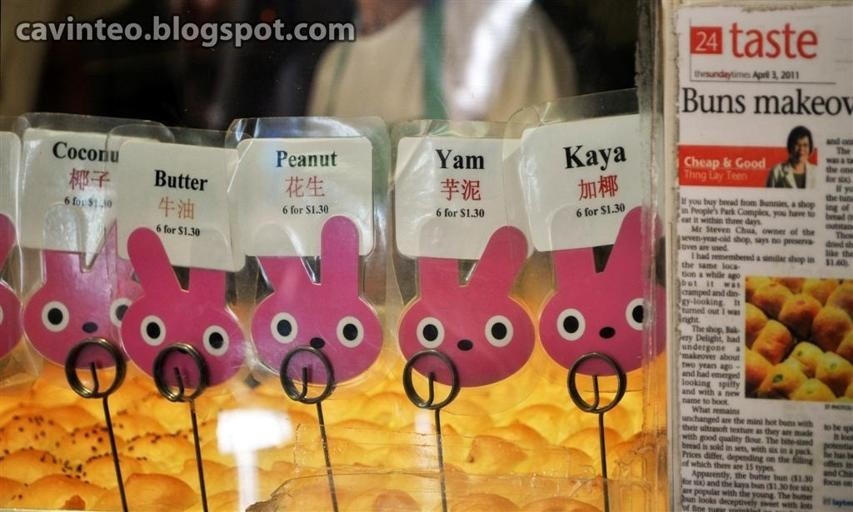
left=745, top=274, right=851, bottom=403
left=1, top=351, right=672, bottom=512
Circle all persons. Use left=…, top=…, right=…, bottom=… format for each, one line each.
left=766, top=126, right=818, bottom=188
left=305, top=0, right=580, bottom=130
